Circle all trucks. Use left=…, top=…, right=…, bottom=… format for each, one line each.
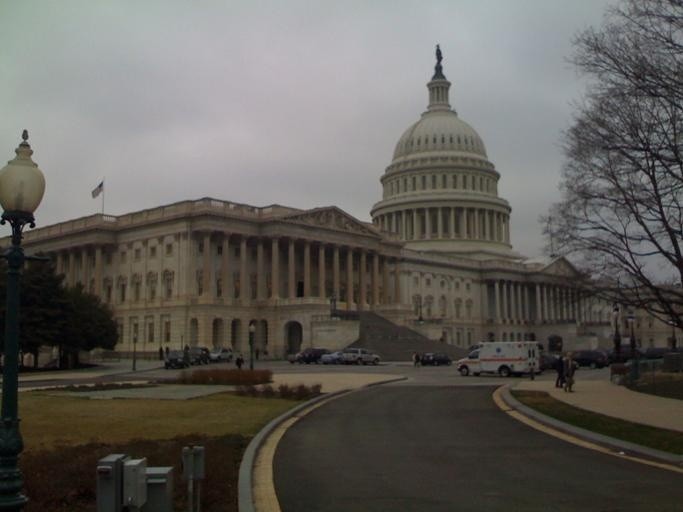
left=457, top=341, right=540, bottom=376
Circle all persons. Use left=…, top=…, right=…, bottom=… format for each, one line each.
left=555, top=351, right=580, bottom=393
left=159, top=345, right=164, bottom=360
left=166, top=344, right=170, bottom=358
left=237, top=354, right=244, bottom=369
left=412, top=350, right=441, bottom=367
left=255, top=347, right=260, bottom=359
left=184, top=343, right=190, bottom=352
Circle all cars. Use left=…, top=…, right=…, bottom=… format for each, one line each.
left=287, top=348, right=381, bottom=367
left=421, top=353, right=451, bottom=366
left=544, top=353, right=578, bottom=369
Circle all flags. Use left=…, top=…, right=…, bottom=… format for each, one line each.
left=91, top=182, right=103, bottom=198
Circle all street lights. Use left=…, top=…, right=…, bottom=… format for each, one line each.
left=611, top=307, right=621, bottom=356
left=131, top=333, right=138, bottom=371
left=0, top=129, right=45, bottom=510
left=248, top=323, right=256, bottom=368
left=625, top=314, right=639, bottom=378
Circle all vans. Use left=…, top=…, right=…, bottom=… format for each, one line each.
left=573, top=349, right=607, bottom=369
left=165, top=346, right=232, bottom=369
